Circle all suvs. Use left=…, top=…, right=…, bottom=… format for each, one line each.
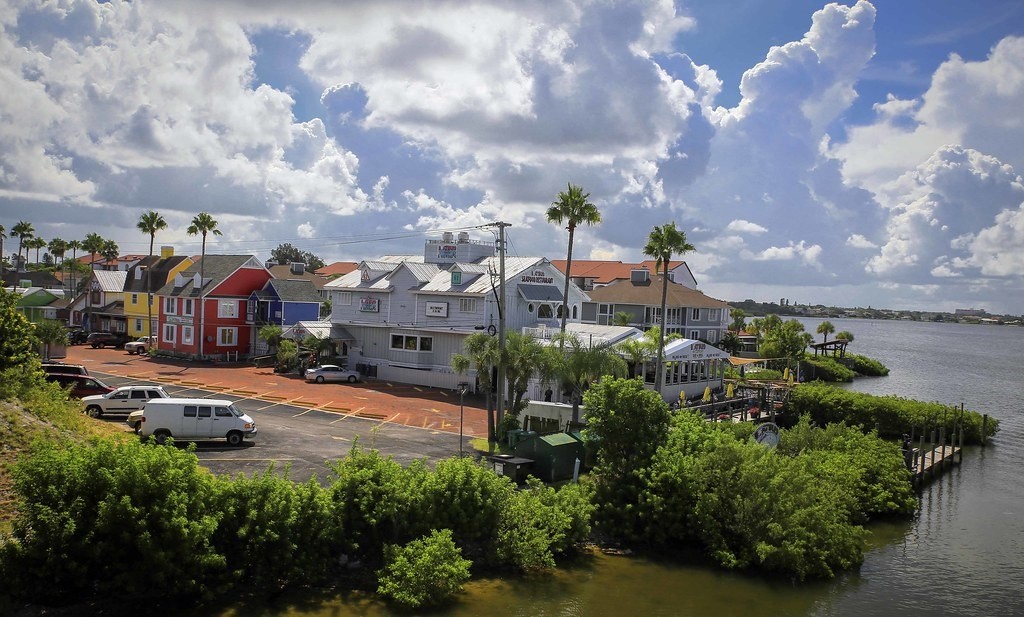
left=65, top=330, right=89, bottom=345
left=124, top=336, right=157, bottom=355
left=80, top=385, right=172, bottom=419
left=45, top=373, right=124, bottom=404
left=87, top=332, right=125, bottom=349
left=40, top=361, right=89, bottom=385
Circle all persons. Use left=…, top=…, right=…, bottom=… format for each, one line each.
left=544, top=386, right=552, bottom=402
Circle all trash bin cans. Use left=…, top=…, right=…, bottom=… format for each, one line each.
left=489, top=429, right=595, bottom=486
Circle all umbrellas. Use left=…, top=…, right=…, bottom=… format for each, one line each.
left=787, top=373, right=794, bottom=387
left=678, top=391, right=686, bottom=407
left=701, top=386, right=711, bottom=403
left=724, top=383, right=733, bottom=398
left=783, top=367, right=788, bottom=381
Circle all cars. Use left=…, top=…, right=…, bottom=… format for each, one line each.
left=305, top=364, right=361, bottom=384
left=126, top=409, right=143, bottom=435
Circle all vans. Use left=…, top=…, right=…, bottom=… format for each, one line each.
left=143, top=397, right=258, bottom=447
